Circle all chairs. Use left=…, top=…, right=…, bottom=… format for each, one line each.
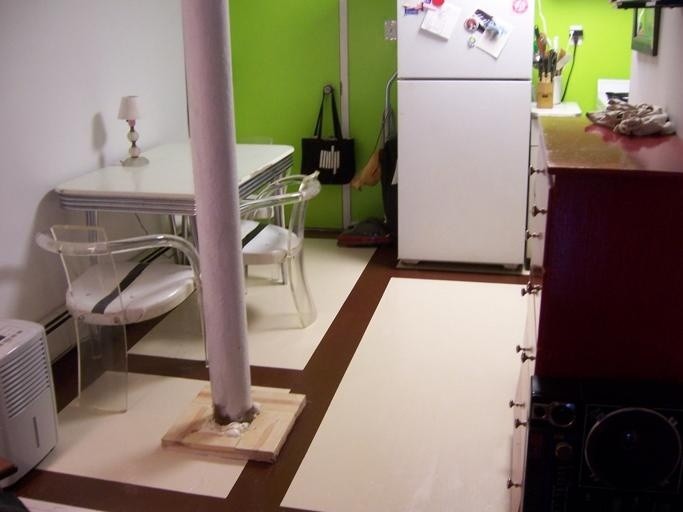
left=236, top=172, right=323, bottom=334
left=37, top=227, right=204, bottom=413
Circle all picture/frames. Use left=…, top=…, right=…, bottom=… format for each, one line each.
left=631, top=7, right=660, bottom=56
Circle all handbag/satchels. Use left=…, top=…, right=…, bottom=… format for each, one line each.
left=300, top=136, right=355, bottom=185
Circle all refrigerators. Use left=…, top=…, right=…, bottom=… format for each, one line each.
left=396, top=0, right=534, bottom=275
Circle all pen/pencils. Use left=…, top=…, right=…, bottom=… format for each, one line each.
left=535, top=30, right=558, bottom=82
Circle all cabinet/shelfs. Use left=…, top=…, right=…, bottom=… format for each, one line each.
left=509, top=113, right=682, bottom=508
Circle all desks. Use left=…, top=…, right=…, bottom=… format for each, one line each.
left=53, top=139, right=296, bottom=336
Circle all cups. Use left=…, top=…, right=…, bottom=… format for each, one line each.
left=553, top=76, right=561, bottom=105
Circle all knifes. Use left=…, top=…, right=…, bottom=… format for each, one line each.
left=538, top=49, right=557, bottom=83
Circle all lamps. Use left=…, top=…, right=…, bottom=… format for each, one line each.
left=117, top=95, right=150, bottom=168
